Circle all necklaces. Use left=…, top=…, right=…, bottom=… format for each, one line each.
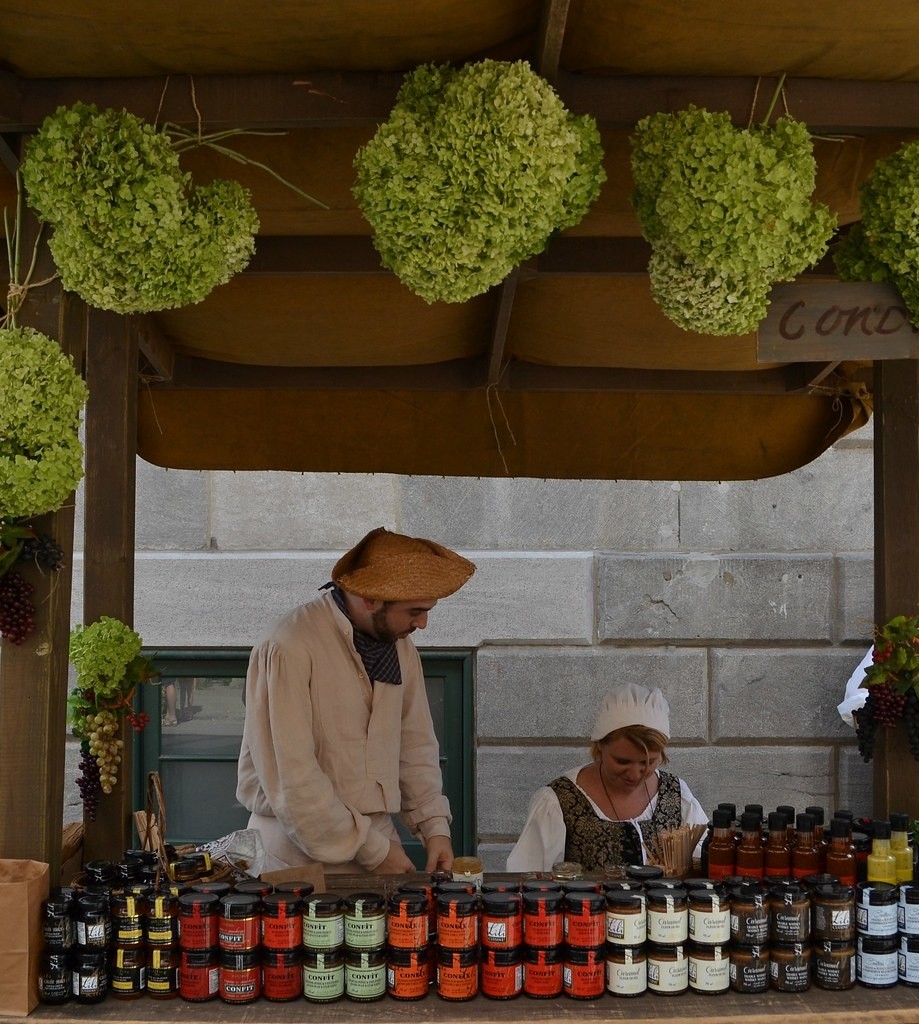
left=600, top=760, right=659, bottom=855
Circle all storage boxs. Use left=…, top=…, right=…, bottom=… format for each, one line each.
left=0, top=858, right=50, bottom=1016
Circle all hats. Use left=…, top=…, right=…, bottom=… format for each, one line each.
left=591, top=683, right=671, bottom=746
left=332, top=526, right=477, bottom=601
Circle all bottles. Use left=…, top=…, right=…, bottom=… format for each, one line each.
left=866, top=821, right=896, bottom=885
left=890, top=812, right=912, bottom=883
left=825, top=809, right=871, bottom=886
left=791, top=806, right=828, bottom=883
left=735, top=804, right=769, bottom=882
left=764, top=806, right=796, bottom=881
left=707, top=803, right=743, bottom=880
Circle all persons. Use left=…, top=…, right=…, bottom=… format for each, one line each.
left=837, top=645, right=874, bottom=729
left=506, top=683, right=736, bottom=873
left=236, top=526, right=477, bottom=875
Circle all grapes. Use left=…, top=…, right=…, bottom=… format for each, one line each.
left=851, top=639, right=919, bottom=762
left=0, top=533, right=65, bottom=645
left=75, top=708, right=124, bottom=824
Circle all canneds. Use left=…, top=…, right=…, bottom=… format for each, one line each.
left=37, top=856, right=919, bottom=1007
